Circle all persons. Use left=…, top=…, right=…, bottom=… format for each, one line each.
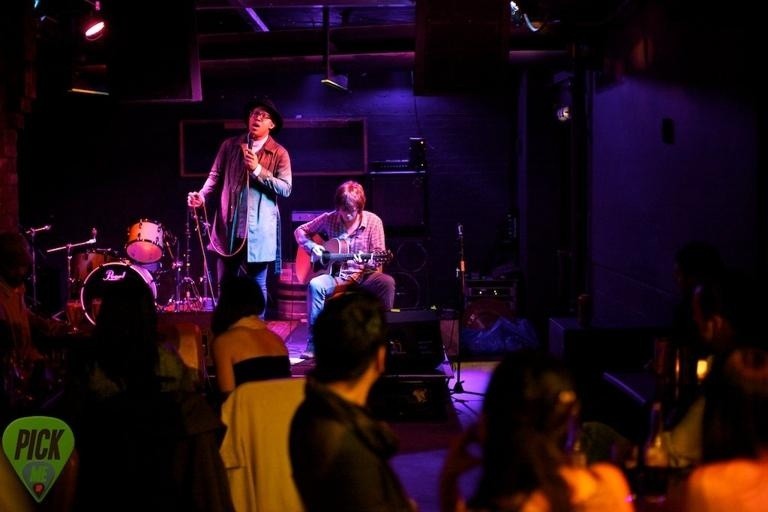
left=185, top=99, right=294, bottom=322
left=293, top=180, right=396, bottom=361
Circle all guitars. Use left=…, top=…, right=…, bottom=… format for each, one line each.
left=81, top=262, right=156, bottom=325
left=295, top=235, right=394, bottom=285
left=126, top=221, right=164, bottom=264
left=76, top=250, right=122, bottom=284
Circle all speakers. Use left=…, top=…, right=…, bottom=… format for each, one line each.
left=369, top=170, right=429, bottom=236
left=380, top=309, right=445, bottom=373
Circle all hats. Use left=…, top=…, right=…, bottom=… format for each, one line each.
left=246, top=98, right=283, bottom=128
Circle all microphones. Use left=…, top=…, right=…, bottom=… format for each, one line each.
left=248, top=132, right=257, bottom=149
left=92, top=228, right=96, bottom=248
left=458, top=225, right=464, bottom=235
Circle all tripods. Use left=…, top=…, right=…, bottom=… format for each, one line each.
left=447, top=238, right=487, bottom=397
left=159, top=208, right=214, bottom=313
left=44, top=239, right=96, bottom=331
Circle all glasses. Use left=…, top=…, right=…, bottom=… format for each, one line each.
left=250, top=111, right=272, bottom=119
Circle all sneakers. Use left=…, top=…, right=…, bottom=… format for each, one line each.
left=300, top=350, right=315, bottom=359
